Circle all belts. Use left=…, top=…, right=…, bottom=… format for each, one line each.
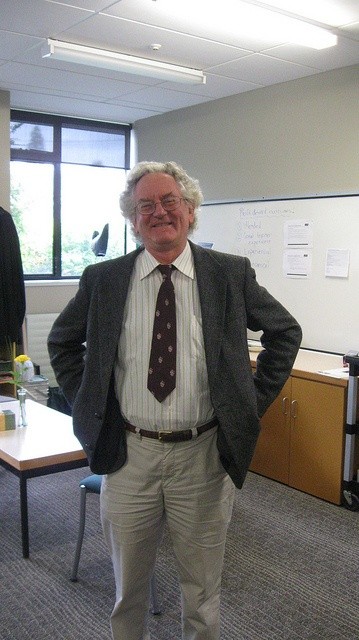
left=124, top=419, right=217, bottom=441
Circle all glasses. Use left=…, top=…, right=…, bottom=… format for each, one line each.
left=134, top=196, right=186, bottom=215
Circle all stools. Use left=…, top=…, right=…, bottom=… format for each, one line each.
left=70, top=474, right=162, bottom=616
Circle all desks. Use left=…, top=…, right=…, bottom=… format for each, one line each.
left=1, top=342, right=106, bottom=559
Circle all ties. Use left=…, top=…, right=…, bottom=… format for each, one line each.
left=147, top=264, right=176, bottom=403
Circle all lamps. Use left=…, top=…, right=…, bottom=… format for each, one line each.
left=39, top=38, right=206, bottom=86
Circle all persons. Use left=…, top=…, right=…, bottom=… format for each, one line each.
left=47, top=161, right=302, bottom=636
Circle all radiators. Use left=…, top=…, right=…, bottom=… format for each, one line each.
left=25, top=312, right=62, bottom=388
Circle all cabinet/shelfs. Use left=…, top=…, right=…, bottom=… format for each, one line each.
left=246, top=339, right=351, bottom=507
left=0, top=340, right=16, bottom=398
